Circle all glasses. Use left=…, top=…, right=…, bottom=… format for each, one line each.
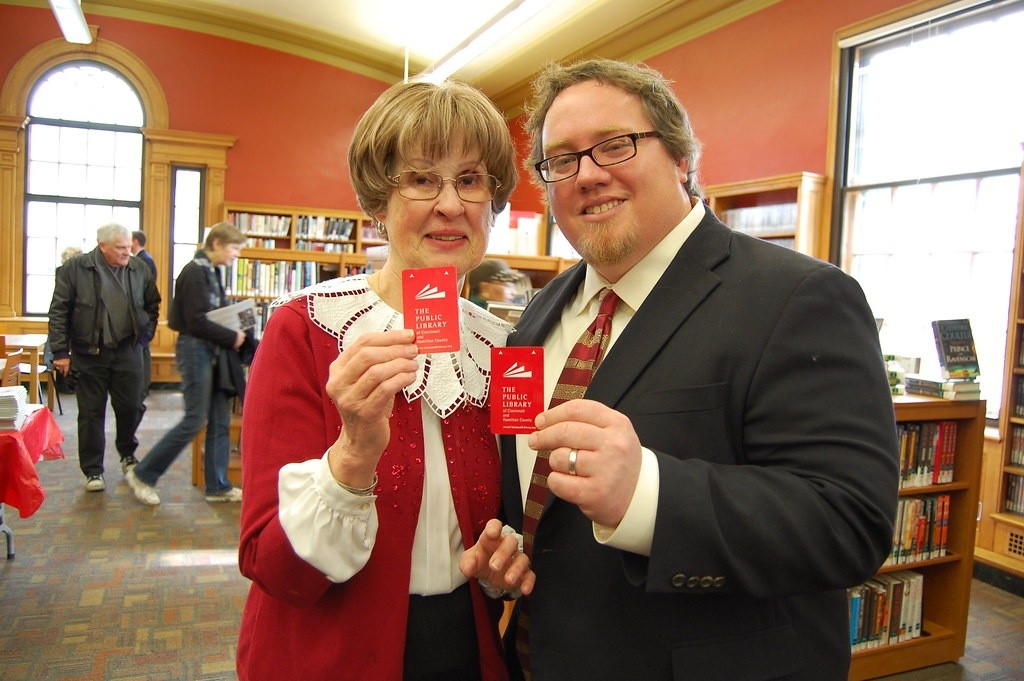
left=384, top=169, right=503, bottom=205
left=535, top=131, right=662, bottom=183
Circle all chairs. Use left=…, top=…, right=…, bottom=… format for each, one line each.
left=1, top=348, right=23, bottom=386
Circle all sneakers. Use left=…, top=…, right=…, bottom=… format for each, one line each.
left=205, top=487, right=242, bottom=503
left=120, top=453, right=139, bottom=475
left=125, top=471, right=161, bottom=505
left=84, top=474, right=106, bottom=491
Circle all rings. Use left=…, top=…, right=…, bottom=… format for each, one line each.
left=569, top=448, right=579, bottom=476
left=56, top=367, right=59, bottom=369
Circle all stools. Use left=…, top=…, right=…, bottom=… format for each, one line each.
left=17, top=363, right=63, bottom=415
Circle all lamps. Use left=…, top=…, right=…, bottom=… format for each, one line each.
left=49, top=0, right=92, bottom=44
left=423, top=0, right=554, bottom=82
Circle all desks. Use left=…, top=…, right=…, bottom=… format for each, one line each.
left=0, top=334, right=56, bottom=411
left=0, top=406, right=65, bottom=558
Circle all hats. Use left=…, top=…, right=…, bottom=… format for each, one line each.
left=468, top=259, right=526, bottom=283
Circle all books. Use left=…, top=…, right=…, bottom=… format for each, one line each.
left=875, top=318, right=981, bottom=401
left=486, top=202, right=583, bottom=260
left=721, top=204, right=799, bottom=251
left=485, top=277, right=542, bottom=326
left=201, top=213, right=389, bottom=466
left=1005, top=322, right=1024, bottom=516
left=0, top=386, right=44, bottom=431
left=846, top=420, right=955, bottom=651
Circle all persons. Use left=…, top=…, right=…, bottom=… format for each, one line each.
left=469, top=259, right=526, bottom=310
left=236, top=82, right=537, bottom=681
left=500, top=62, right=898, bottom=681
left=47, top=225, right=246, bottom=505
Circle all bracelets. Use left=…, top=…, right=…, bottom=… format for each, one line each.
left=336, top=474, right=378, bottom=496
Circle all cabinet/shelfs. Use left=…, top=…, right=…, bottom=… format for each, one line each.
left=191, top=201, right=373, bottom=488
left=847, top=395, right=986, bottom=681
left=982, top=161, right=1024, bottom=580
left=465, top=253, right=579, bottom=318
left=696, top=171, right=828, bottom=262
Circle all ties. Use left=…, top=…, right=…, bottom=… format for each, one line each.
left=512, top=289, right=619, bottom=680
